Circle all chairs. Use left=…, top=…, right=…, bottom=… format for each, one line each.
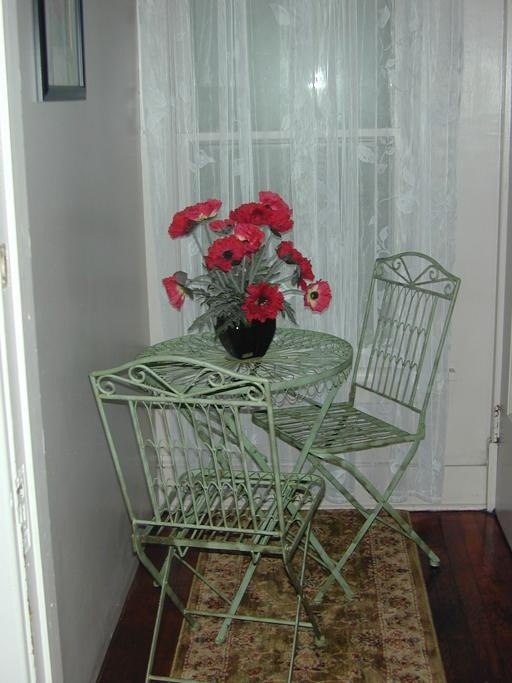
left=88, top=354, right=326, bottom=683
left=251, top=252, right=461, bottom=605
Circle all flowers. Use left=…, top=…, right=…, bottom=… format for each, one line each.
left=161, top=190, right=332, bottom=343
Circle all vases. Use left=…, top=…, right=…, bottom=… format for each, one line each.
left=213, top=314, right=277, bottom=360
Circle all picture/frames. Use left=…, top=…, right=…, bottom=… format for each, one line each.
left=31, top=1, right=87, bottom=103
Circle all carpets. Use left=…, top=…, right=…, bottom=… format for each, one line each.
left=165, top=510, right=446, bottom=683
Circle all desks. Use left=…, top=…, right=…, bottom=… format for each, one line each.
left=136, top=325, right=356, bottom=643
